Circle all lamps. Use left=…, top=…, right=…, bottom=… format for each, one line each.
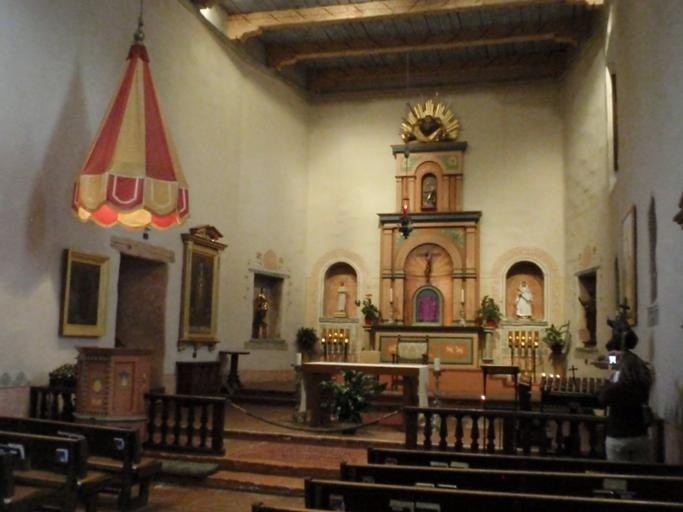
left=69, top=0, right=190, bottom=232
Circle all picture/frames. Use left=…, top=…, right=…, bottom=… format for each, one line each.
left=180, top=224, right=227, bottom=345
left=614, top=205, right=639, bottom=328
left=59, top=249, right=111, bottom=339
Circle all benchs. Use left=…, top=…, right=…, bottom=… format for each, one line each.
left=0, top=414, right=164, bottom=511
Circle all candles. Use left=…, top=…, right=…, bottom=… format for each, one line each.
left=389, top=288, right=393, bottom=302
left=460, top=288, right=465, bottom=302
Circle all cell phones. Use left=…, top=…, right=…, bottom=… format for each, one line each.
left=609, top=354, right=617, bottom=367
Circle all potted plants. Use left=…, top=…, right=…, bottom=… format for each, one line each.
left=541, top=321, right=572, bottom=356
left=312, top=365, right=385, bottom=437
left=361, top=296, right=378, bottom=324
left=476, top=295, right=502, bottom=329
left=295, top=326, right=318, bottom=362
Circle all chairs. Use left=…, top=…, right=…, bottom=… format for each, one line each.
left=388, top=334, right=430, bottom=394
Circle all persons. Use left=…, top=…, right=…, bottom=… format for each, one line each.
left=595, top=341, right=654, bottom=465
left=514, top=279, right=534, bottom=320
left=335, top=282, right=349, bottom=313
left=253, top=284, right=270, bottom=338
